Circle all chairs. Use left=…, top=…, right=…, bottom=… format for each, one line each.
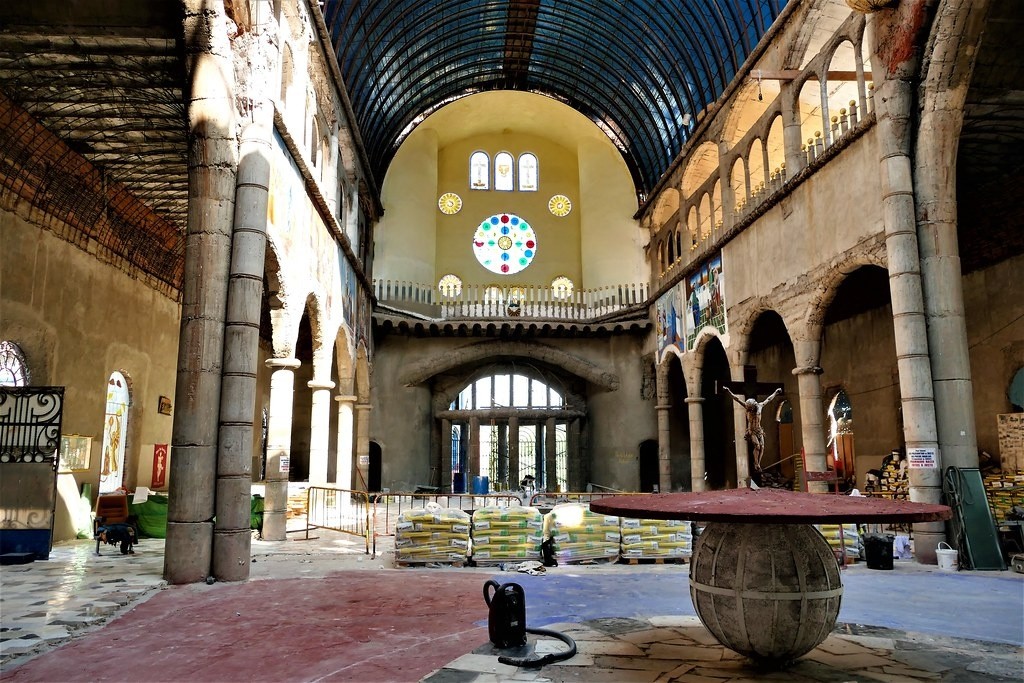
left=91, top=494, right=134, bottom=557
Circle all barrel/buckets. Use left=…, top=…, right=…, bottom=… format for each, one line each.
left=454, top=472, right=467, bottom=494
left=860, top=532, right=896, bottom=571
left=473, top=476, right=488, bottom=494
left=935, top=541, right=958, bottom=570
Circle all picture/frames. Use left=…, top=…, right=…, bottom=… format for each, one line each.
left=57, top=434, right=95, bottom=474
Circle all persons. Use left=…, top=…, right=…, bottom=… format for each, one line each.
left=722, top=385, right=781, bottom=483
